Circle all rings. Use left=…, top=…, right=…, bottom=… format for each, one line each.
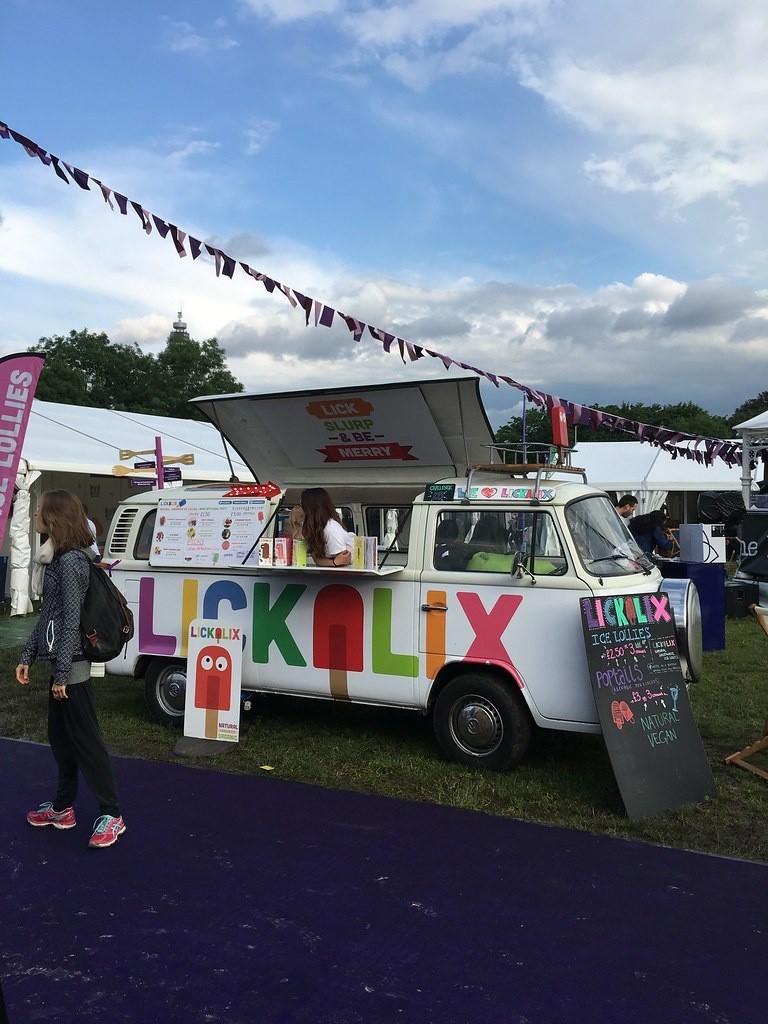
left=54, top=695, right=58, bottom=699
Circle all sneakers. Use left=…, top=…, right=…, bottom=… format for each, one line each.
left=27, top=801, right=77, bottom=829
left=88, top=814, right=127, bottom=848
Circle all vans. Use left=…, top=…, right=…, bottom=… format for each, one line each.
left=96, top=376, right=704, bottom=766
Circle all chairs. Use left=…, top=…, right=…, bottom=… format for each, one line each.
left=467, top=515, right=510, bottom=559
left=723, top=603, right=768, bottom=783
left=434, top=519, right=467, bottom=571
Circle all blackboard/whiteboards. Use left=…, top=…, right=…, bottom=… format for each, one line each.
left=580, top=592, right=719, bottom=822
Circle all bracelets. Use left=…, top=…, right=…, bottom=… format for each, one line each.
left=332, top=558, right=338, bottom=567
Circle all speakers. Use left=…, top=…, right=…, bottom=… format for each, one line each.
left=726, top=581, right=760, bottom=619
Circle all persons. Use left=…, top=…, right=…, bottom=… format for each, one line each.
left=80, top=503, right=101, bottom=564
left=14, top=490, right=127, bottom=848
left=300, top=487, right=355, bottom=567
left=615, top=494, right=638, bottom=518
left=626, top=510, right=672, bottom=566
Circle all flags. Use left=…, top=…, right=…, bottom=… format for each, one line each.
left=397, top=338, right=767, bottom=472
left=0, top=120, right=395, bottom=354
left=1, top=351, right=46, bottom=554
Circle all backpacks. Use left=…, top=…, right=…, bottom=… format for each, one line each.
left=55, top=547, right=135, bottom=663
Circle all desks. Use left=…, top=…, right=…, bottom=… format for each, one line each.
left=656, top=559, right=725, bottom=652
